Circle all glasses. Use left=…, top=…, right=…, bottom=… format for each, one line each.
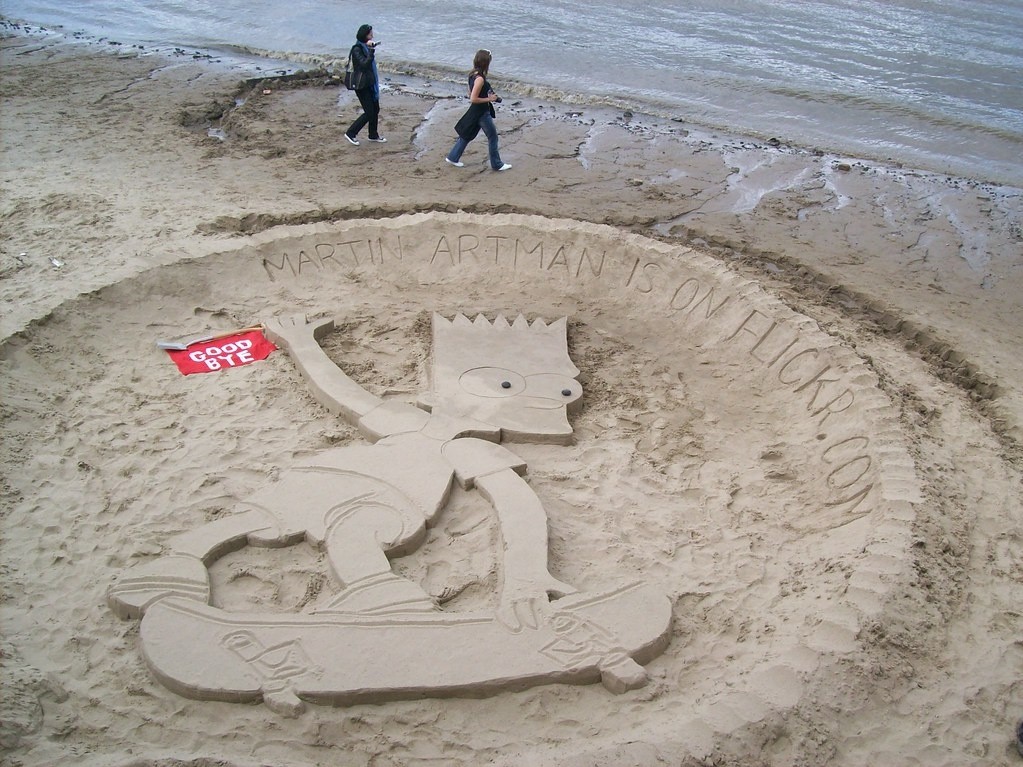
left=480, top=48, right=492, bottom=57
left=362, top=25, right=373, bottom=31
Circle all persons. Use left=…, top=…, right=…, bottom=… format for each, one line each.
left=106, top=305, right=604, bottom=629
left=341, top=23, right=388, bottom=148
left=446, top=49, right=512, bottom=171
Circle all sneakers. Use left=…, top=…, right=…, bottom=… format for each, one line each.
left=495, top=163, right=513, bottom=171
left=367, top=134, right=388, bottom=144
left=445, top=157, right=464, bottom=167
left=343, top=132, right=360, bottom=147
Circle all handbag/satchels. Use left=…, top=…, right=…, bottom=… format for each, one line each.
left=345, top=71, right=367, bottom=91
